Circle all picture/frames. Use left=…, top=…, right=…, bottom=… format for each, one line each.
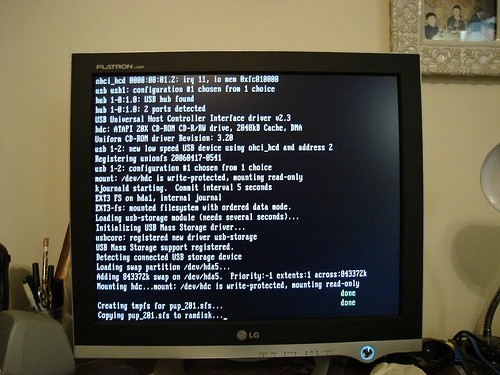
left=390, top=0, right=500, bottom=76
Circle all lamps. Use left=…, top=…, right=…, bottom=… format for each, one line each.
left=465, top=143, right=500, bottom=375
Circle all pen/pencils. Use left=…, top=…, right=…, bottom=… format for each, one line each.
left=22, top=220, right=70, bottom=313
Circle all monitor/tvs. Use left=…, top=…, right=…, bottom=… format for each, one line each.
left=70, top=52, right=424, bottom=364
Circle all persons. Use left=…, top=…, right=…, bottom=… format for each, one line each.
left=425, top=12, right=439, bottom=39
left=447, top=4, right=465, bottom=30
left=469, top=6, right=484, bottom=23
